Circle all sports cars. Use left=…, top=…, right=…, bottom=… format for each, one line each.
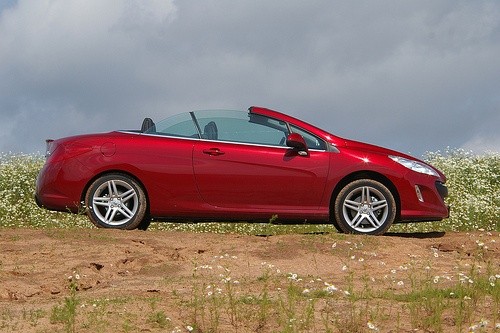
left=35, top=106, right=449, bottom=236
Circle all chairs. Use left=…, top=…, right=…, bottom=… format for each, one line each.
left=204, top=121, right=218, bottom=139
left=141, top=118, right=156, bottom=133
